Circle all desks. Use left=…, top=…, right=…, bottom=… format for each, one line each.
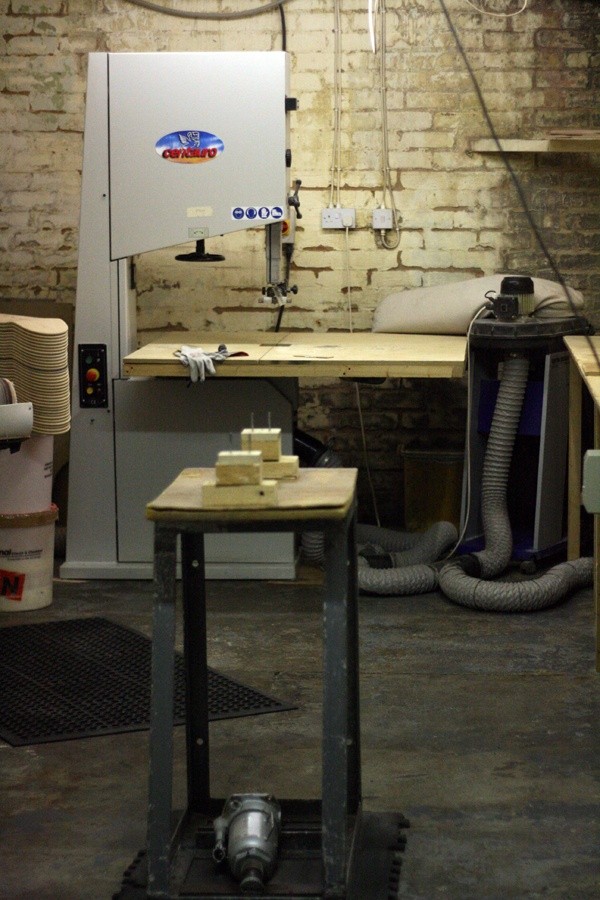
left=563, top=332, right=600, bottom=640
left=122, top=332, right=471, bottom=379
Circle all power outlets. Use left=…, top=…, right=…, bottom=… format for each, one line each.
left=372, top=209, right=393, bottom=229
left=323, top=208, right=356, bottom=229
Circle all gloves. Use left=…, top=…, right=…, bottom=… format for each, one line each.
left=181, top=345, right=226, bottom=384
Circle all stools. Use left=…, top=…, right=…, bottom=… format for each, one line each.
left=143, top=466, right=363, bottom=896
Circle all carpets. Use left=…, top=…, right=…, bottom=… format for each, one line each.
left=112, top=810, right=411, bottom=900
